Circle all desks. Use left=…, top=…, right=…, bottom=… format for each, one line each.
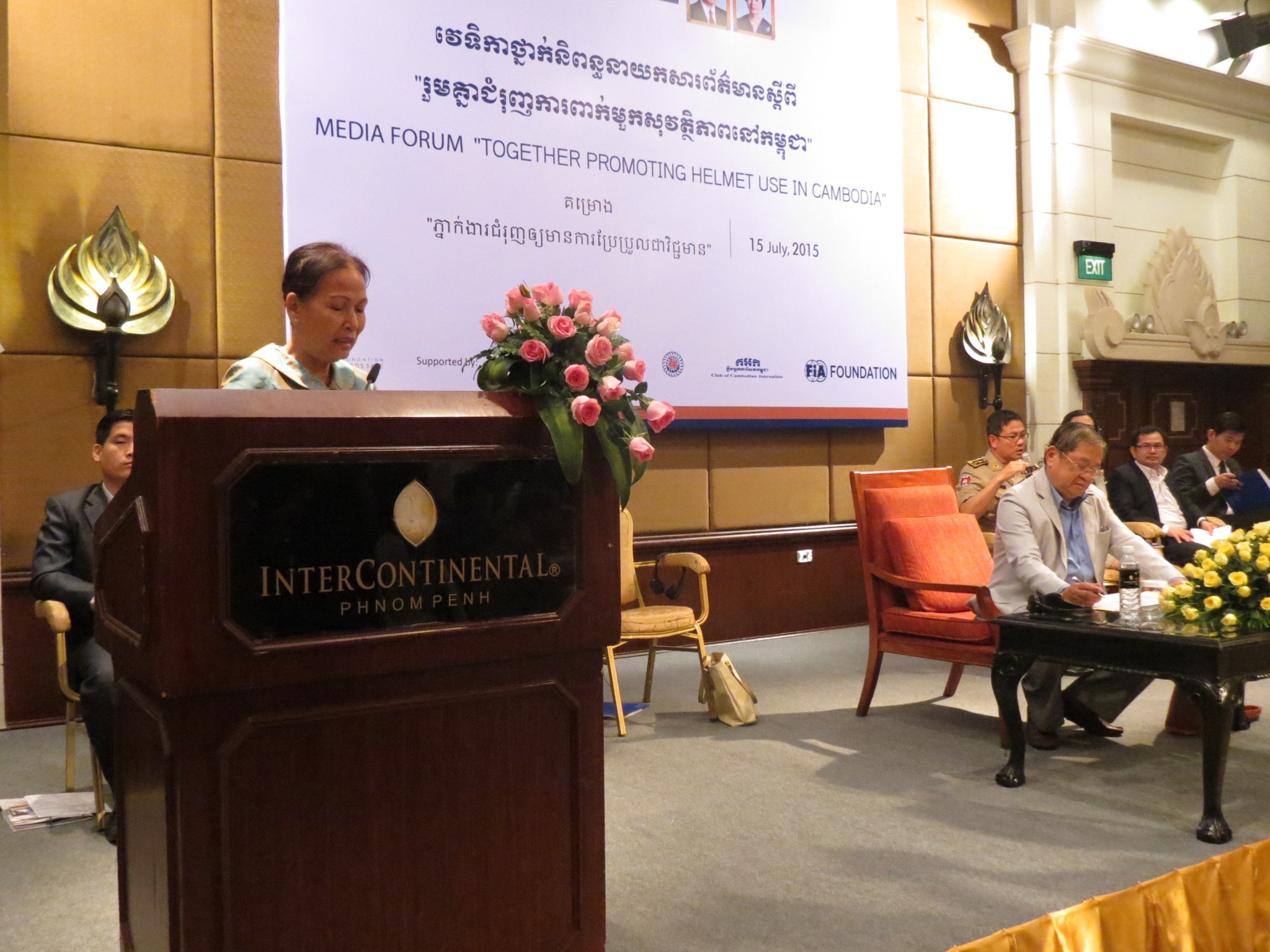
left=990, top=597, right=1270, bottom=843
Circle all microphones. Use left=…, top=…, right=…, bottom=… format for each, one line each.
left=364, top=363, right=381, bottom=391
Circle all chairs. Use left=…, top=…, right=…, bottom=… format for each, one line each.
left=848, top=464, right=1007, bottom=745
left=603, top=503, right=717, bottom=735
left=34, top=594, right=109, bottom=829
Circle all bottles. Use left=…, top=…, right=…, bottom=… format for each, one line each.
left=1022, top=452, right=1040, bottom=479
left=1119, top=546, right=1141, bottom=626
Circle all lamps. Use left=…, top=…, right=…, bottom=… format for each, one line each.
left=1221, top=319, right=1248, bottom=337
left=1124, top=313, right=1156, bottom=333
left=1222, top=1, right=1270, bottom=59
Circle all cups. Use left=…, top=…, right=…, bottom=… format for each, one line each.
left=1138, top=585, right=1168, bottom=630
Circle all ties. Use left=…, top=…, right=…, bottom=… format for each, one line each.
left=1220, top=460, right=1239, bottom=515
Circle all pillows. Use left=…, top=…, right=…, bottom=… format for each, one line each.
left=884, top=510, right=999, bottom=614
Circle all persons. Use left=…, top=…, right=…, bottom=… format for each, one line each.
left=956, top=409, right=1040, bottom=555
left=1062, top=410, right=1099, bottom=430
left=222, top=243, right=375, bottom=391
left=970, top=421, right=1187, bottom=750
left=30, top=409, right=134, bottom=842
left=736, top=0, right=772, bottom=36
left=689, top=0, right=726, bottom=25
left=1106, top=424, right=1217, bottom=565
left=1171, top=411, right=1247, bottom=531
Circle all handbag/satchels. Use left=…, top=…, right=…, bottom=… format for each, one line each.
left=698, top=653, right=758, bottom=725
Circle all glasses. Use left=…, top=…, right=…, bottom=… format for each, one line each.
left=1060, top=450, right=1100, bottom=478
left=1135, top=442, right=1163, bottom=451
left=995, top=432, right=1029, bottom=442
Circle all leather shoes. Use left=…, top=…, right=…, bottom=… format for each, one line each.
left=104, top=808, right=119, bottom=844
left=1027, top=706, right=1061, bottom=750
left=1061, top=690, right=1124, bottom=736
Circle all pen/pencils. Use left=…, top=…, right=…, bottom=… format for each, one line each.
left=1072, top=577, right=1106, bottom=599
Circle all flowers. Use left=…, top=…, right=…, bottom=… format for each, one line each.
left=1159, top=519, right=1270, bottom=629
left=461, top=280, right=679, bottom=511
